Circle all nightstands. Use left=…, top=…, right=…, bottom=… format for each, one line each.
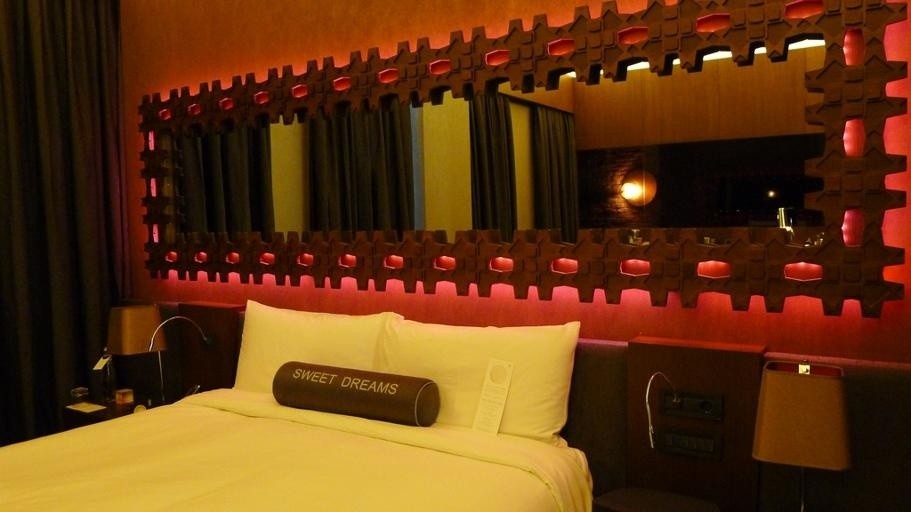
left=63, top=398, right=176, bottom=427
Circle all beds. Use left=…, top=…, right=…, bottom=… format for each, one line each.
left=0, top=304, right=631, bottom=511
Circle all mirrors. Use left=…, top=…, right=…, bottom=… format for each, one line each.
left=138, top=1, right=908, bottom=321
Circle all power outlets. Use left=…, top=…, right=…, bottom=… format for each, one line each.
left=655, top=388, right=726, bottom=460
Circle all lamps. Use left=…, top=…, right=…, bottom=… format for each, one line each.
left=752, top=361, right=854, bottom=511
left=105, top=303, right=170, bottom=402
